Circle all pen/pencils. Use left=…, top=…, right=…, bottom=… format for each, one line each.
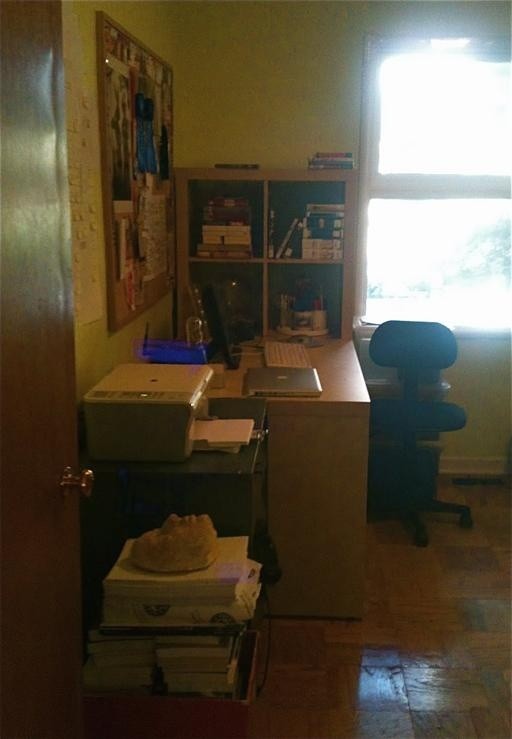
left=280, top=293, right=296, bottom=308
left=313, top=294, right=327, bottom=310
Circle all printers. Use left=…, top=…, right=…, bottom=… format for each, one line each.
left=81, top=362, right=255, bottom=462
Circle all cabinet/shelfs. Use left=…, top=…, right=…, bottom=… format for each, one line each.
left=78, top=396, right=279, bottom=693
left=174, top=166, right=358, bottom=337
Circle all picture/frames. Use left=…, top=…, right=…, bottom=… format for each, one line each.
left=96, top=10, right=174, bottom=330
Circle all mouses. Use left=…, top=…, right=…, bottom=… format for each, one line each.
left=288, top=333, right=313, bottom=345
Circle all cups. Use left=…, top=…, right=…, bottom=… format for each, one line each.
left=311, top=310, right=328, bottom=330
left=278, top=307, right=294, bottom=329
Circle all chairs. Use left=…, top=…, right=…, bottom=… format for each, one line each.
left=370, top=321, right=473, bottom=547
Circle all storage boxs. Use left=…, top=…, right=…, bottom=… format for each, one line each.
left=82, top=630, right=259, bottom=739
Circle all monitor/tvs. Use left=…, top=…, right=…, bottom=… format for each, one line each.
left=200, top=278, right=244, bottom=369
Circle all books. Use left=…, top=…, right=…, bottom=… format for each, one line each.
left=196, top=196, right=254, bottom=259
left=307, top=152, right=355, bottom=170
left=301, top=203, right=344, bottom=260
left=86, top=628, right=242, bottom=695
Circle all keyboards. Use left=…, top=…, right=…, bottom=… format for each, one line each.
left=264, top=339, right=312, bottom=367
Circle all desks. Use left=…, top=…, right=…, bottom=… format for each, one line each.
left=137, top=336, right=372, bottom=621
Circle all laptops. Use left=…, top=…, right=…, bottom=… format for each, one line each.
left=245, top=366, right=322, bottom=397
